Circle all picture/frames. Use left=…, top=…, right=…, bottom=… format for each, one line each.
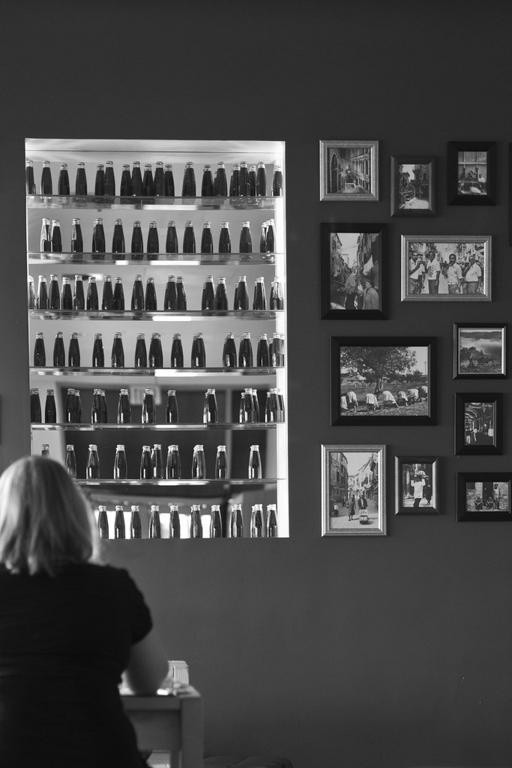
left=329, top=336, right=438, bottom=426
left=456, top=472, right=512, bottom=522
left=393, top=455, right=440, bottom=515
left=390, top=155, right=438, bottom=217
left=400, top=233, right=493, bottom=303
left=318, top=138, right=381, bottom=202
left=320, top=222, right=390, bottom=320
left=452, top=322, right=507, bottom=380
left=320, top=443, right=387, bottom=538
left=446, top=141, right=497, bottom=206
left=453, top=392, right=504, bottom=457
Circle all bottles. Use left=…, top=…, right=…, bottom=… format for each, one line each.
left=28, top=273, right=282, bottom=315
left=39, top=443, right=262, bottom=483
left=34, top=331, right=282, bottom=370
left=26, top=160, right=282, bottom=203
left=96, top=505, right=277, bottom=539
left=30, top=385, right=283, bottom=426
left=37, top=217, right=275, bottom=262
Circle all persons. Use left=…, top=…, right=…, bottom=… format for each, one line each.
left=409, top=250, right=483, bottom=296
left=492, top=483, right=502, bottom=509
left=474, top=493, right=483, bottom=509
left=409, top=474, right=426, bottom=506
left=0, top=453, right=168, bottom=768
left=362, top=280, right=379, bottom=310
left=358, top=493, right=368, bottom=510
left=340, top=386, right=428, bottom=412
left=485, top=496, right=494, bottom=509
left=345, top=497, right=357, bottom=519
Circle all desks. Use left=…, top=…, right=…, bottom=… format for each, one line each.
left=119, top=685, right=205, bottom=768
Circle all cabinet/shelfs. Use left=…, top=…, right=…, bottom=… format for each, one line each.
left=23, top=137, right=290, bottom=540
